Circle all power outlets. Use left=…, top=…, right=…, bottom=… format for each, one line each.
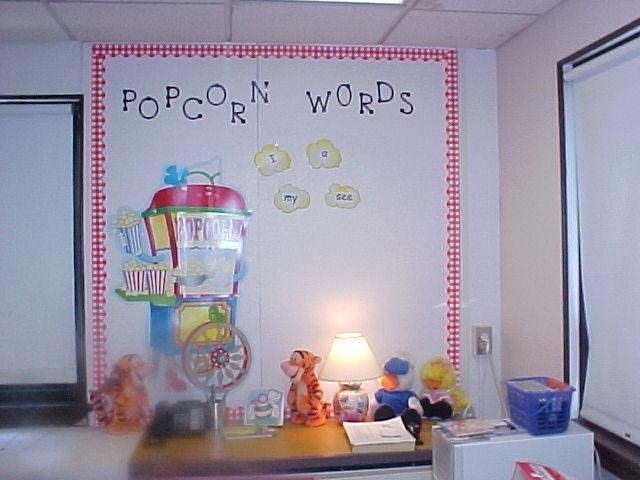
left=470, top=325, right=493, bottom=356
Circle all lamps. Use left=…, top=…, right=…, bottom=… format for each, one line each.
left=317, top=334, right=388, bottom=425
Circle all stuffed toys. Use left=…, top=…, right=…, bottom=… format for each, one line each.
left=279, top=348, right=328, bottom=428
left=89, top=353, right=151, bottom=433
left=414, top=353, right=471, bottom=417
left=373, top=355, right=425, bottom=421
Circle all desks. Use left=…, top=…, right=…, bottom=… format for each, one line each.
left=130, top=407, right=436, bottom=480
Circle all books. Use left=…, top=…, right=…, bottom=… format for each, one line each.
left=440, top=418, right=495, bottom=441
left=342, top=414, right=417, bottom=453
left=241, top=389, right=286, bottom=427
left=221, top=423, right=273, bottom=440
